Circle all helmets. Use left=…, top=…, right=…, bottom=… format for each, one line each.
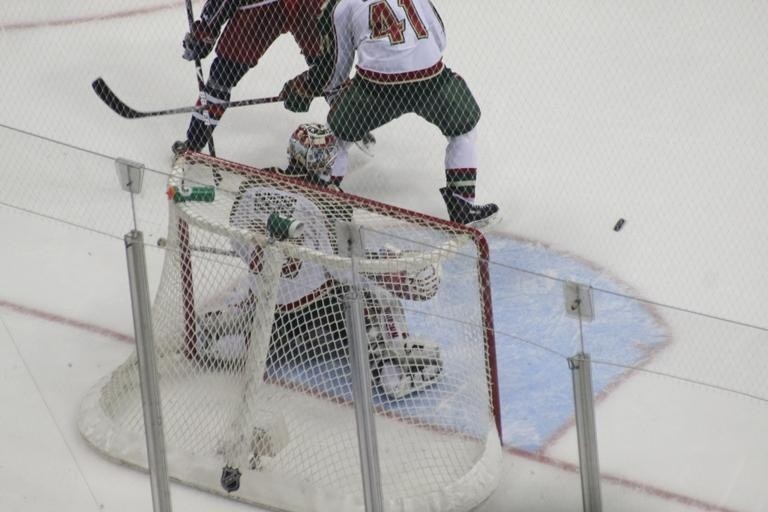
left=287, top=123, right=336, bottom=168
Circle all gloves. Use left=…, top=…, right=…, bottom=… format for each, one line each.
left=279, top=79, right=314, bottom=113
left=181, top=20, right=220, bottom=60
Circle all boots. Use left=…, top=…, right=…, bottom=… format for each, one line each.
left=441, top=187, right=499, bottom=226
left=172, top=140, right=202, bottom=166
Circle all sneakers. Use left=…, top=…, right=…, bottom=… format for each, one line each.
left=378, top=337, right=447, bottom=398
left=192, top=309, right=226, bottom=367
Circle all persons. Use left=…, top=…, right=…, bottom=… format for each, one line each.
left=277, top=0, right=499, bottom=226
left=168, top=0, right=376, bottom=166
left=167, top=121, right=445, bottom=400
